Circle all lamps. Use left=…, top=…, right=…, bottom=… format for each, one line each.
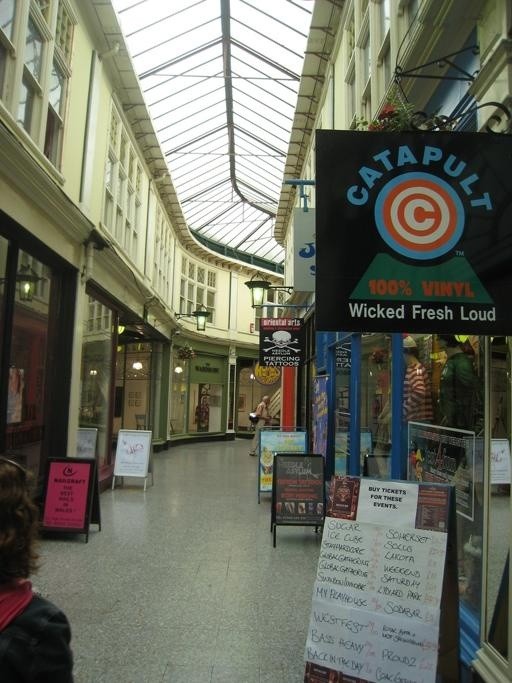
left=174, top=303, right=211, bottom=330
left=0, top=262, right=46, bottom=302
left=244, top=271, right=293, bottom=308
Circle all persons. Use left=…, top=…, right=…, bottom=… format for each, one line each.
left=1, top=455, right=75, bottom=682
left=437, top=333, right=484, bottom=456
left=370, top=351, right=393, bottom=481
left=402, top=335, right=435, bottom=482
left=249, top=394, right=272, bottom=456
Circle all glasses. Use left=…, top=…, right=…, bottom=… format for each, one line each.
left=0, top=453, right=27, bottom=481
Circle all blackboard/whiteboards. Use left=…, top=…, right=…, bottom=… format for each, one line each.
left=113, top=429, right=153, bottom=478
left=75, top=427, right=98, bottom=459
